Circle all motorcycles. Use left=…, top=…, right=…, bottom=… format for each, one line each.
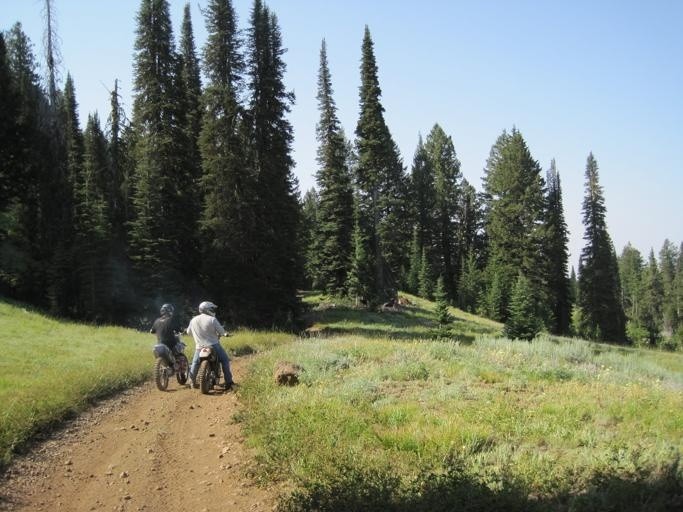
left=153, top=330, right=190, bottom=392
left=199, top=333, right=231, bottom=393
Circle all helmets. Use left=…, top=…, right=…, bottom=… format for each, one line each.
left=160, top=303, right=174, bottom=314
left=199, top=301, right=218, bottom=317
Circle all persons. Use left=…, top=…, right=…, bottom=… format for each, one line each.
left=150, top=304, right=186, bottom=381
left=186, top=300, right=238, bottom=391
